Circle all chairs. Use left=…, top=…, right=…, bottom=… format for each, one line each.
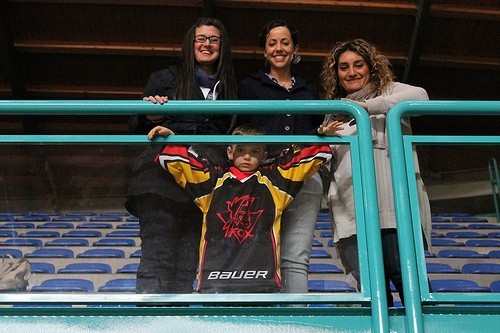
left=0, top=210, right=500, bottom=306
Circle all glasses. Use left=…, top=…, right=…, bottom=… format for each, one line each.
left=192, top=34, right=222, bottom=44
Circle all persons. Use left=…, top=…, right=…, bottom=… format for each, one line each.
left=148, top=115, right=345, bottom=307
left=319, top=38, right=433, bottom=307
left=238, top=20, right=331, bottom=308
left=123, top=16, right=238, bottom=307
